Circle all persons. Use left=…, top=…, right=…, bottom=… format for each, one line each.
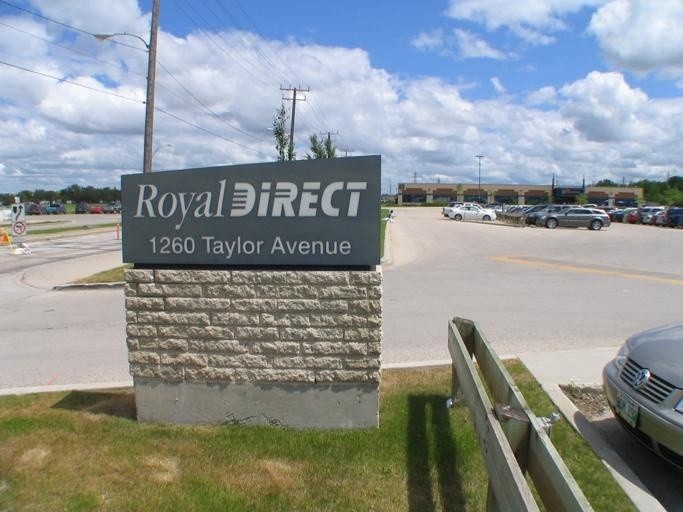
left=387, top=210, right=393, bottom=223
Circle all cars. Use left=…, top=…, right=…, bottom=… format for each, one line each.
left=602, top=323, right=682, bottom=480
left=24, top=200, right=121, bottom=214
left=441, top=201, right=683, bottom=231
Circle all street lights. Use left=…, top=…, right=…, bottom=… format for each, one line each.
left=475, top=154, right=484, bottom=202
left=94, top=31, right=156, bottom=174
left=153, top=144, right=172, bottom=159
